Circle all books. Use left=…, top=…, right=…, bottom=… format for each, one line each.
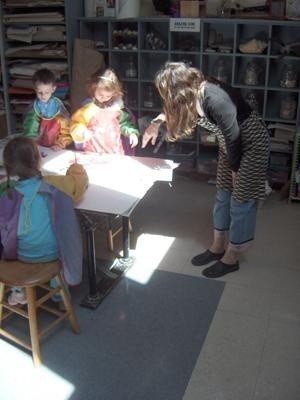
left=257, top=120, right=296, bottom=183
left=0, top=0, right=74, bottom=134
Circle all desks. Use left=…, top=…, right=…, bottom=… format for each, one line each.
left=0, top=139, right=157, bottom=311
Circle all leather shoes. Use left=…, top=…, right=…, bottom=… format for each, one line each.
left=191, top=249, right=225, bottom=266
left=202, top=260, right=239, bottom=278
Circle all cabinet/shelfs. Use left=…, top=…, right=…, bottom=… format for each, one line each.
left=0, top=1, right=84, bottom=135
left=79, top=17, right=300, bottom=183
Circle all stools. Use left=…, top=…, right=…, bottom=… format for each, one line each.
left=0, top=260, right=82, bottom=367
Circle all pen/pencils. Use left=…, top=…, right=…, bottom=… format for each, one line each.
left=75, top=154, right=77, bottom=164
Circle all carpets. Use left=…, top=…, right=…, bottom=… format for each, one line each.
left=0, top=256, right=227, bottom=399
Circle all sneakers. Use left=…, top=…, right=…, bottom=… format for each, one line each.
left=58, top=300, right=66, bottom=312
left=8, top=289, right=27, bottom=305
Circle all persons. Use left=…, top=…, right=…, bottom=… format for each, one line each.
left=141, top=60, right=272, bottom=279
left=0, top=135, right=89, bottom=311
left=21, top=68, right=74, bottom=153
left=67, top=67, right=140, bottom=156
